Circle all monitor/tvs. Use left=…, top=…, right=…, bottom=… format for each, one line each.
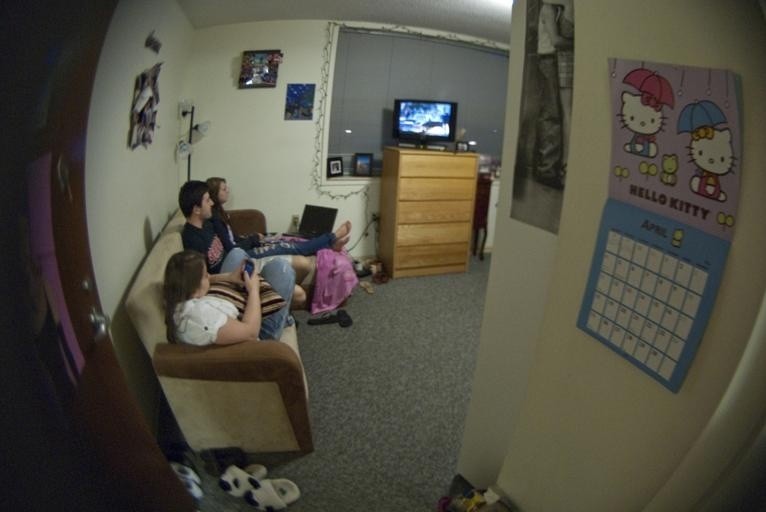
left=392, top=99, right=458, bottom=151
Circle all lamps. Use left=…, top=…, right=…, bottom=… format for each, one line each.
left=177, top=106, right=210, bottom=181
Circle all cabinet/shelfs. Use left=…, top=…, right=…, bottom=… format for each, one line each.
left=378, top=145, right=479, bottom=280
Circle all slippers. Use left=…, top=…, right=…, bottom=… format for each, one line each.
left=244, top=478, right=300, bottom=512
left=218, top=464, right=268, bottom=497
left=337, top=310, right=350, bottom=327
left=373, top=271, right=388, bottom=285
left=359, top=281, right=375, bottom=294
left=308, top=312, right=338, bottom=325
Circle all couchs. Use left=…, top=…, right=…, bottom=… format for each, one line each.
left=124, top=208, right=314, bottom=458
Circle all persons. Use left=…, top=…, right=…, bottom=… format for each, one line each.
left=178, top=180, right=312, bottom=312
left=205, top=176, right=352, bottom=259
left=162, top=250, right=296, bottom=346
left=511, top=1, right=576, bottom=196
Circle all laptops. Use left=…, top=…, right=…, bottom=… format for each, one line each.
left=282, top=204, right=338, bottom=239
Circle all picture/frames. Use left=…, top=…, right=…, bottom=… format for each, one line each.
left=353, top=152, right=374, bottom=177
left=327, top=157, right=343, bottom=178
left=239, top=49, right=281, bottom=88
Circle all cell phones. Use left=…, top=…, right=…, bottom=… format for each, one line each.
left=240, top=259, right=254, bottom=282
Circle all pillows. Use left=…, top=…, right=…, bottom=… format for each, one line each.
left=206, top=274, right=287, bottom=323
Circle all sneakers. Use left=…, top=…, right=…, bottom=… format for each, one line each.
left=164, top=442, right=203, bottom=500
left=353, top=258, right=383, bottom=276
left=200, top=446, right=249, bottom=476
